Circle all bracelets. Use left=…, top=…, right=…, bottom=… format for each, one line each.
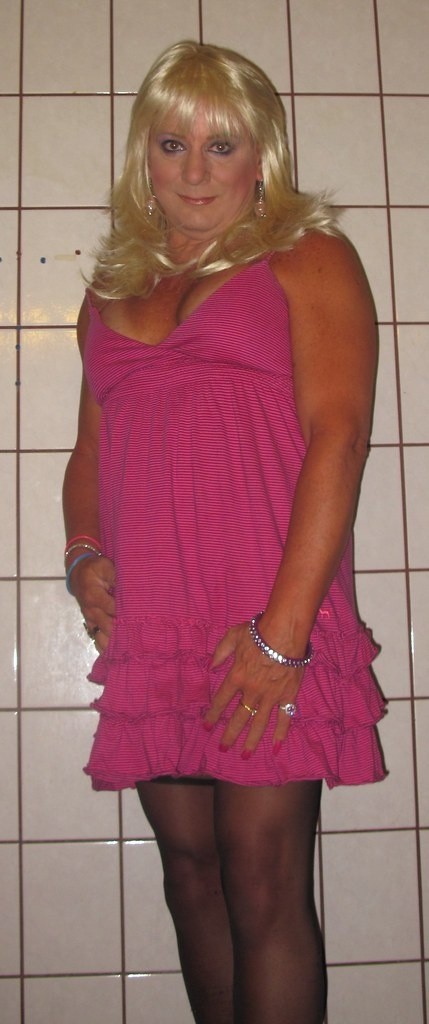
left=64, top=543, right=101, bottom=569
left=65, top=535, right=101, bottom=557
left=248, top=611, right=314, bottom=669
left=65, top=552, right=97, bottom=595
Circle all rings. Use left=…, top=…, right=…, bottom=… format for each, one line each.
left=84, top=623, right=100, bottom=640
left=278, top=703, right=297, bottom=716
left=239, top=699, right=257, bottom=716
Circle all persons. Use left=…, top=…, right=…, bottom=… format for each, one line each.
left=63, top=41, right=389, bottom=1024
